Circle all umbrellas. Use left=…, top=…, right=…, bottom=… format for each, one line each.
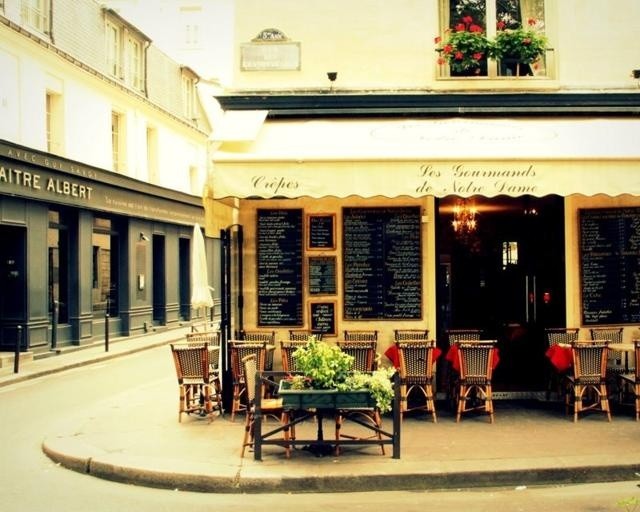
left=191, top=222, right=214, bottom=332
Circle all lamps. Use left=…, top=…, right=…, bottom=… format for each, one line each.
left=452, top=197, right=480, bottom=232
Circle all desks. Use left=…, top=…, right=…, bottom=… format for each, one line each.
left=278, top=378, right=374, bottom=458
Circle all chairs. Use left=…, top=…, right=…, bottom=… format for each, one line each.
left=331, top=354, right=407, bottom=459
left=279, top=341, right=316, bottom=373
left=393, top=339, right=437, bottom=426
left=288, top=328, right=324, bottom=387
left=455, top=338, right=496, bottom=424
left=446, top=329, right=484, bottom=347
left=241, top=354, right=295, bottom=461
left=236, top=330, right=275, bottom=398
left=335, top=340, right=378, bottom=373
left=545, top=327, right=640, bottom=422
left=393, top=328, right=434, bottom=417
left=226, top=338, right=268, bottom=421
left=170, top=341, right=222, bottom=421
left=344, top=329, right=379, bottom=372
left=185, top=333, right=221, bottom=394
